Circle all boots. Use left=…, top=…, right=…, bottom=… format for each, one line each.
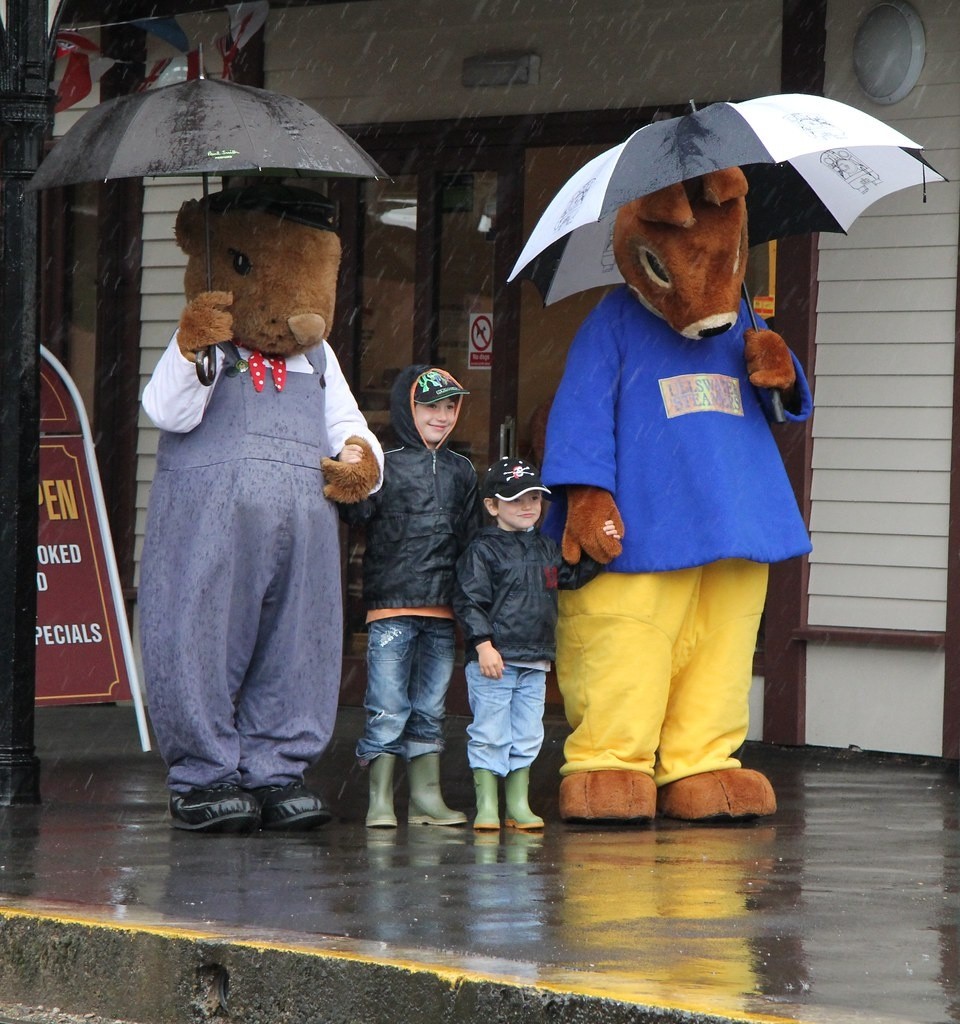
left=473, top=769, right=500, bottom=828
left=504, top=768, right=545, bottom=829
left=365, top=754, right=397, bottom=827
left=407, top=753, right=468, bottom=825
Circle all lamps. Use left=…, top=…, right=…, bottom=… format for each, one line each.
left=851, top=1, right=925, bottom=106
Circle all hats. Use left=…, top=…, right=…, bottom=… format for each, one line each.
left=414, top=371, right=470, bottom=405
left=478, top=457, right=552, bottom=502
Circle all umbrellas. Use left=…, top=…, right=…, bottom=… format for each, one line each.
left=505, top=91, right=951, bottom=423
left=19, top=44, right=395, bottom=387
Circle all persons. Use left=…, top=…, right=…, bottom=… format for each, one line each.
left=331, top=366, right=619, bottom=830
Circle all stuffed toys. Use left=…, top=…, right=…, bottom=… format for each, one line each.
left=140, top=172, right=384, bottom=830
left=540, top=167, right=812, bottom=821
